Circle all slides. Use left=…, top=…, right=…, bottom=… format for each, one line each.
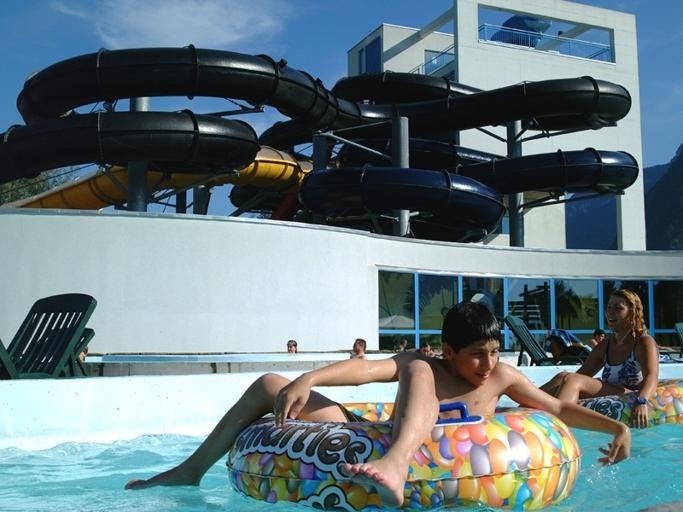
left=0, top=45, right=639, bottom=242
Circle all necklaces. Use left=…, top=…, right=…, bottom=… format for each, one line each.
left=613, top=326, right=631, bottom=345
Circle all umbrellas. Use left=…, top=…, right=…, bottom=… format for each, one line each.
left=378, top=313, right=416, bottom=330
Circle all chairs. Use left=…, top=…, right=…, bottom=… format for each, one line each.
left=0, top=292, right=97, bottom=377
left=505, top=315, right=592, bottom=366
left=674, top=321, right=683, bottom=359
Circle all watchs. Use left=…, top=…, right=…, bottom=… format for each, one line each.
left=634, top=395, right=649, bottom=407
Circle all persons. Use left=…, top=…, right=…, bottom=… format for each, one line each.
left=411, top=342, right=435, bottom=357
left=287, top=340, right=298, bottom=353
left=518, top=289, right=661, bottom=430
left=123, top=297, right=631, bottom=509
left=588, top=328, right=605, bottom=350
left=348, top=339, right=367, bottom=361
left=390, top=337, right=407, bottom=353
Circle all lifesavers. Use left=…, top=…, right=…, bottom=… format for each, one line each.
left=226, top=402, right=582, bottom=510
left=579, top=377, right=683, bottom=429
